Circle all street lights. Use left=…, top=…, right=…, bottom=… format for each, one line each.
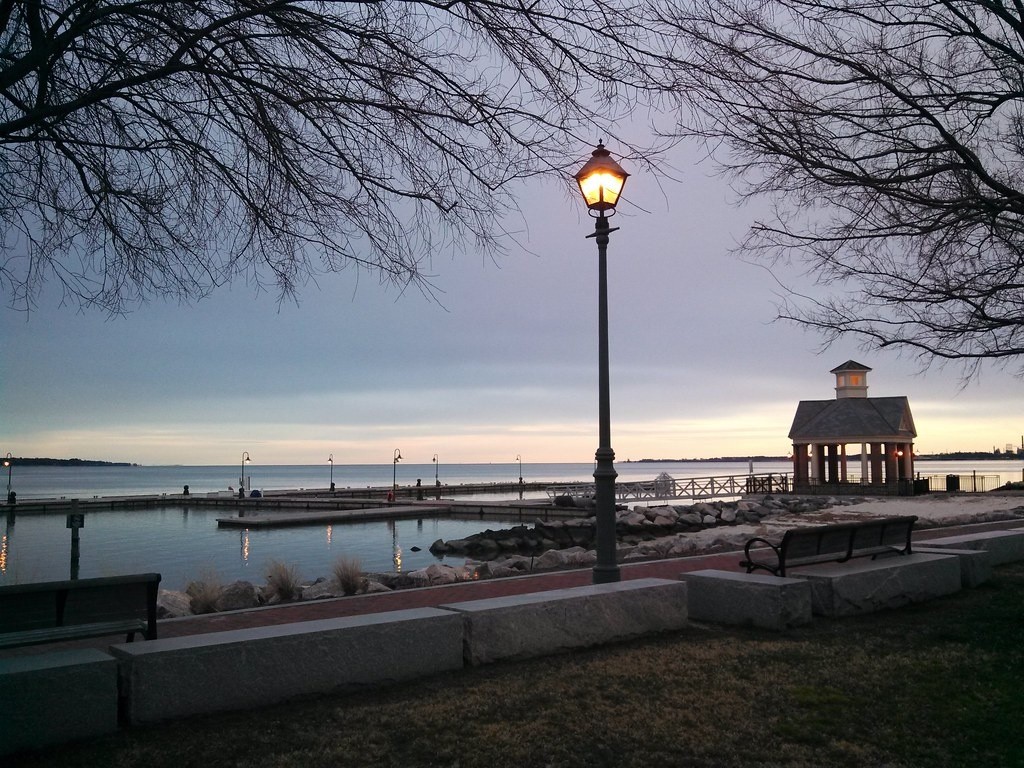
left=328, top=454, right=335, bottom=491
left=517, top=455, right=522, bottom=483
left=4, top=452, right=12, bottom=504
left=432, top=454, right=439, bottom=487
left=392, top=448, right=404, bottom=502
left=239, top=450, right=252, bottom=499
left=574, top=137, right=629, bottom=584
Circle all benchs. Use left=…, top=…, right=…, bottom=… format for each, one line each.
left=739, top=515, right=918, bottom=578
left=0, top=573, right=162, bottom=651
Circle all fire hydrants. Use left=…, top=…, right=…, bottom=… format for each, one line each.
left=417, top=479, right=421, bottom=487
